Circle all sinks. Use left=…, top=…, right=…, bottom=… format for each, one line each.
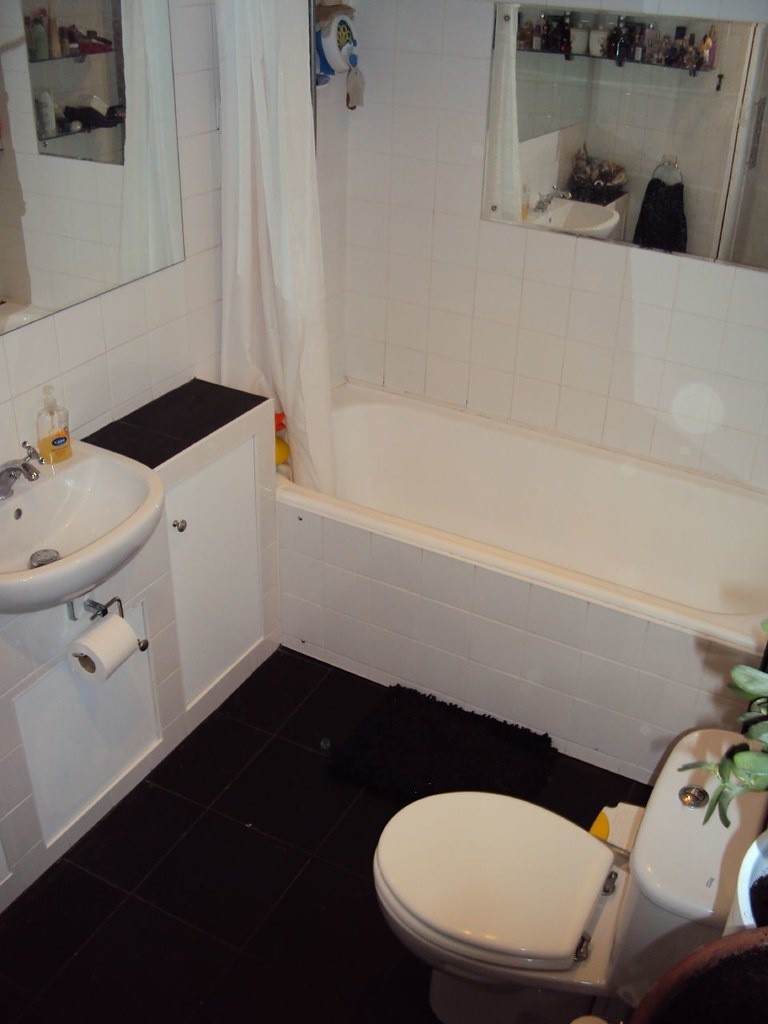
left=516, top=193, right=620, bottom=239
left=0, top=440, right=166, bottom=617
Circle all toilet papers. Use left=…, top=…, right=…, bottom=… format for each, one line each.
left=65, top=612, right=138, bottom=685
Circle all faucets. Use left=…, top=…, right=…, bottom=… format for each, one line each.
left=0, top=458, right=40, bottom=502
left=540, top=185, right=570, bottom=212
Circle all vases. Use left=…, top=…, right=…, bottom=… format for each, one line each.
left=635, top=928, right=768, bottom=1024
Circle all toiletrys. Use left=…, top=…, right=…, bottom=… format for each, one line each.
left=36, top=382, right=73, bottom=465
left=520, top=173, right=531, bottom=220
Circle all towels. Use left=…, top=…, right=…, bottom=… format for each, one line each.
left=632, top=177, right=688, bottom=256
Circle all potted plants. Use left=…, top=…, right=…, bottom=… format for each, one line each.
left=675, top=619, right=768, bottom=938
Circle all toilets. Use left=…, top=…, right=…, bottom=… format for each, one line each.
left=371, top=726, right=768, bottom=1023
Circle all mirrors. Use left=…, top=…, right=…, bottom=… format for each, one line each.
left=0, top=0, right=189, bottom=336
left=478, top=0, right=768, bottom=273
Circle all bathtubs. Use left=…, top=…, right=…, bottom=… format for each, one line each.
left=277, top=381, right=768, bottom=789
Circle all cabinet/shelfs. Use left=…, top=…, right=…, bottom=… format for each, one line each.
left=32, top=45, right=123, bottom=141
left=0, top=376, right=284, bottom=914
